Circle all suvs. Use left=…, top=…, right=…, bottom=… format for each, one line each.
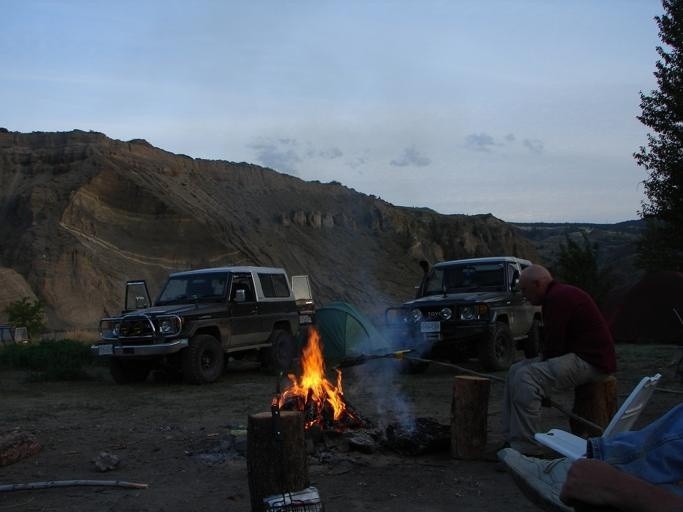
left=89, top=265, right=317, bottom=387
left=0, top=324, right=32, bottom=349
left=384, top=256, right=543, bottom=372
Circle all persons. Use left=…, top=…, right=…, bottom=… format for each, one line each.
left=489, top=263, right=616, bottom=455
left=494, top=400, right=682, bottom=511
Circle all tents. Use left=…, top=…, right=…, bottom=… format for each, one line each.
left=295, top=302, right=388, bottom=363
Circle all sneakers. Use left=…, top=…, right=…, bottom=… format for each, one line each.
left=496, top=448, right=574, bottom=512
